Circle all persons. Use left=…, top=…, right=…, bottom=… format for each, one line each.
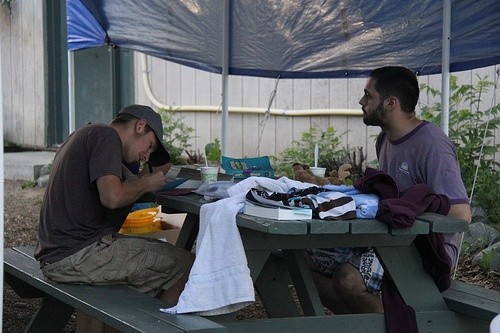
left=32, top=103, right=195, bottom=305
left=304, top=67, right=472, bottom=314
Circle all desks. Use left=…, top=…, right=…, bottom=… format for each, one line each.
left=163, top=193, right=470, bottom=333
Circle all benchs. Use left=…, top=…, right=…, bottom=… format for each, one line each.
left=4, top=245, right=499, bottom=333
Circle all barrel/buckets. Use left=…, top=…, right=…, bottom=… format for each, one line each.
left=119, top=208, right=159, bottom=234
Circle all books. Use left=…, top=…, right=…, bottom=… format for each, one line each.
left=176, top=155, right=313, bottom=221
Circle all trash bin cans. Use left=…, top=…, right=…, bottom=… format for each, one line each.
left=122, top=208, right=160, bottom=234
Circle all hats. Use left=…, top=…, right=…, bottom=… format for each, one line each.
left=116, top=104, right=171, bottom=167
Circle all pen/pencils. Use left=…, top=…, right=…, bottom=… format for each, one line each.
left=148, top=160, right=153, bottom=174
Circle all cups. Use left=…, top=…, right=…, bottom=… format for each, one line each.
left=310, top=167, right=326, bottom=177
left=200, top=167, right=218, bottom=183
